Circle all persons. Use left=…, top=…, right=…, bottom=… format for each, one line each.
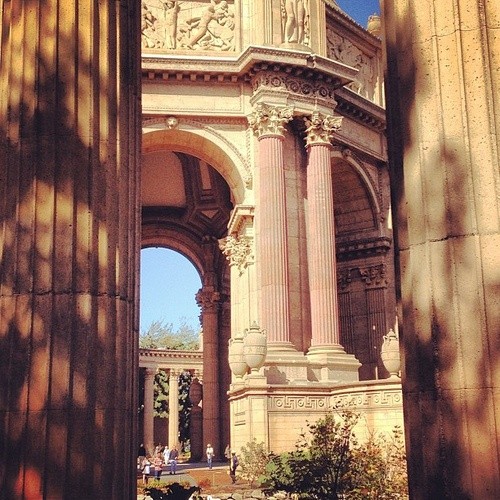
left=137, top=442, right=240, bottom=485
left=285, top=0, right=306, bottom=44
left=141, top=0, right=236, bottom=51
